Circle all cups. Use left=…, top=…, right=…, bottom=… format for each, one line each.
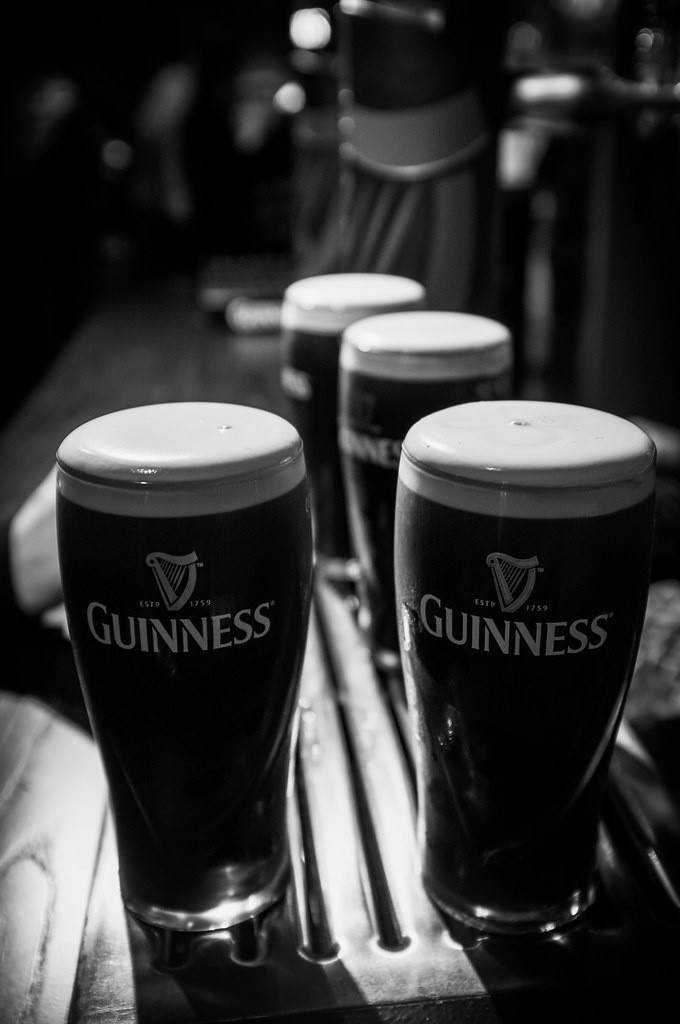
left=394, top=401, right=658, bottom=934
left=55, top=403, right=314, bottom=931
left=339, top=310, right=513, bottom=671
left=280, top=273, right=424, bottom=575
